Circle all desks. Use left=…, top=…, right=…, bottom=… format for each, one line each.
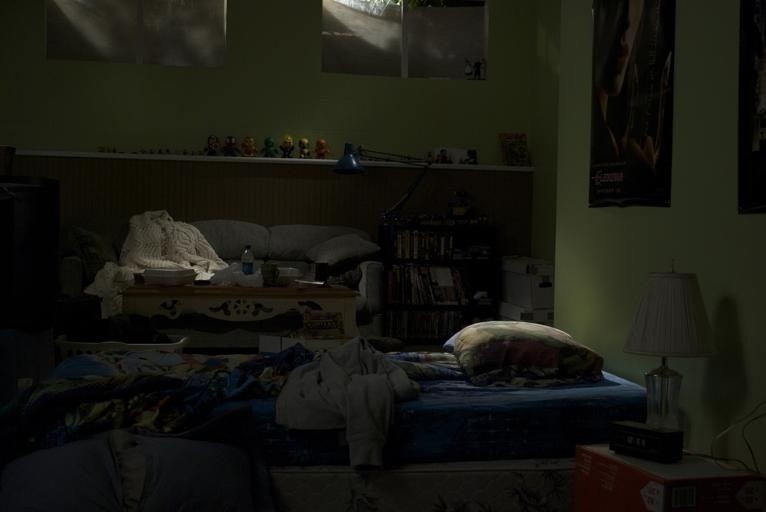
left=103, top=274, right=363, bottom=367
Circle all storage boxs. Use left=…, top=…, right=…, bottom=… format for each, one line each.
left=500, top=302, right=553, bottom=328
left=502, top=253, right=555, bottom=310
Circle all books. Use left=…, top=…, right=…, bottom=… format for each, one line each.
left=387, top=227, right=472, bottom=344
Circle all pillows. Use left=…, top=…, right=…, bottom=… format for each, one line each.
left=441, top=315, right=606, bottom=389
left=303, top=230, right=379, bottom=265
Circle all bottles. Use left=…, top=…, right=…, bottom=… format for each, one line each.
left=241, top=243, right=254, bottom=274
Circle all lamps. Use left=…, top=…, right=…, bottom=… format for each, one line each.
left=331, top=138, right=436, bottom=244
left=624, top=269, right=711, bottom=431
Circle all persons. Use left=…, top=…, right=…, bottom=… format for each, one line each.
left=458, top=149, right=479, bottom=165
left=473, top=59, right=481, bottom=79
left=481, top=57, right=487, bottom=79
left=436, top=148, right=453, bottom=164
left=591, top=1, right=658, bottom=169
left=463, top=59, right=473, bottom=80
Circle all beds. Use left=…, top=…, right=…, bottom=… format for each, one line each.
left=0, top=317, right=653, bottom=510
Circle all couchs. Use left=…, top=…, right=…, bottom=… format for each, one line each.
left=83, top=217, right=385, bottom=355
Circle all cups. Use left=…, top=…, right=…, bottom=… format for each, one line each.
left=314, top=260, right=328, bottom=281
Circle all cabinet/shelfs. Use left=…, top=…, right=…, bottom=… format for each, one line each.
left=388, top=216, right=503, bottom=350
left=567, top=442, right=763, bottom=511
